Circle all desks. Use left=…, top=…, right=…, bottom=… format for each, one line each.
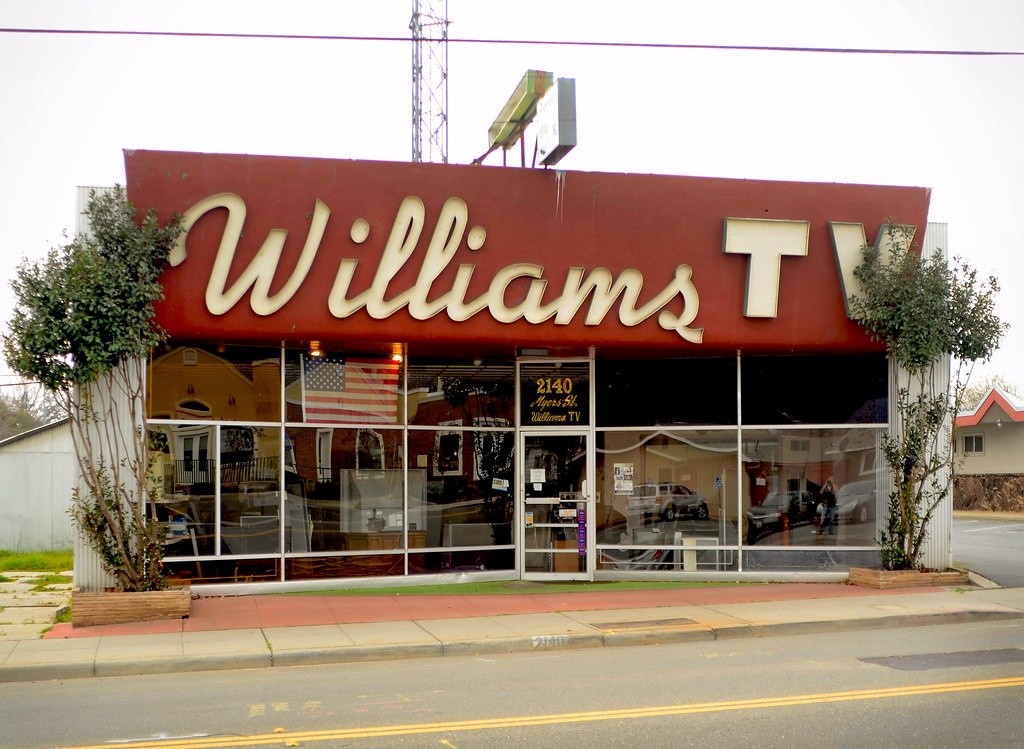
left=340, top=531, right=428, bottom=550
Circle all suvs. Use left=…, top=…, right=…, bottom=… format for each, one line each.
left=625, top=481, right=709, bottom=522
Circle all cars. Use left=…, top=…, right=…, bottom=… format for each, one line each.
left=746, top=490, right=808, bottom=530
left=816, top=480, right=876, bottom=524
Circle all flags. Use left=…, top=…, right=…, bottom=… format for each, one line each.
left=300, top=353, right=399, bottom=425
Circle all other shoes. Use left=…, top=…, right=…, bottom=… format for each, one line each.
left=816, top=533, right=823, bottom=535
left=829, top=533, right=833, bottom=534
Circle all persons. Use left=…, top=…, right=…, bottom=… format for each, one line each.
left=816, top=476, right=839, bottom=538
